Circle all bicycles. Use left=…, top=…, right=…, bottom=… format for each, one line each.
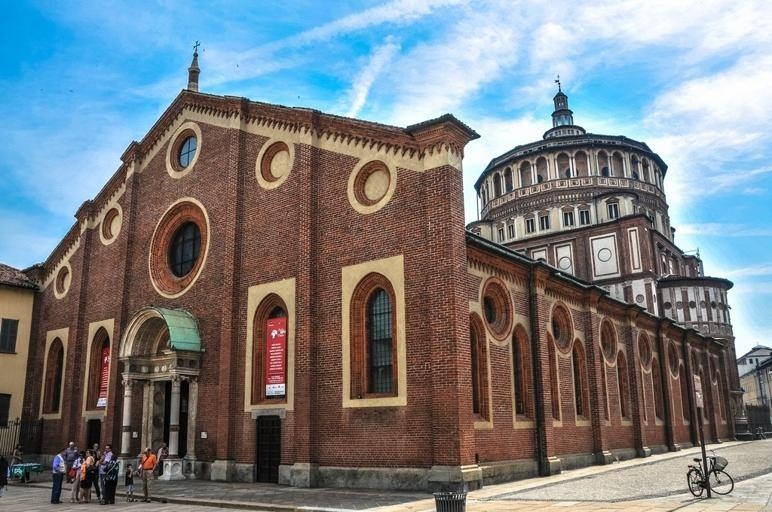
left=685, top=449, right=734, bottom=498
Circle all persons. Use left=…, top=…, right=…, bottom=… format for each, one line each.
left=50, top=442, right=119, bottom=504
left=0, top=455, right=10, bottom=497
left=138, top=448, right=157, bottom=503
left=157, top=443, right=168, bottom=475
left=10, top=444, right=23, bottom=464
left=125, top=464, right=139, bottom=502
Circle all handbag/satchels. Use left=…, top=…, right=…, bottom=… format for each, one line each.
left=68, top=459, right=79, bottom=478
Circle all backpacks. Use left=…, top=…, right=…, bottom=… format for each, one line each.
left=85, top=465, right=98, bottom=481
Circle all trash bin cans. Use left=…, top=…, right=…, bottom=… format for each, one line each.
left=432, top=492, right=467, bottom=512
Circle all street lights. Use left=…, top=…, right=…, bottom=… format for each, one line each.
left=680, top=328, right=712, bottom=498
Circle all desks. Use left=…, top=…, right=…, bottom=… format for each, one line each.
left=9, top=463, right=44, bottom=486
